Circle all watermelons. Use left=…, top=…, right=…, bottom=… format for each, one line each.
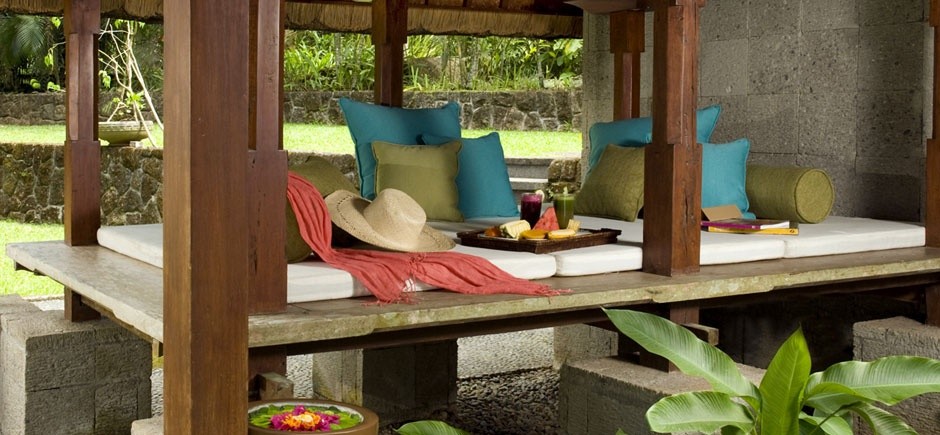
left=533, top=207, right=559, bottom=232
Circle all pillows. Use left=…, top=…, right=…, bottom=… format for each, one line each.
left=339, top=96, right=521, bottom=222
left=574, top=104, right=835, bottom=223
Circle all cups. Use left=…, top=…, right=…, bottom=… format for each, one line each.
left=520, top=193, right=542, bottom=230
left=553, top=194, right=576, bottom=229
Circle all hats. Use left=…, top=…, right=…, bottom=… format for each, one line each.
left=324, top=188, right=456, bottom=253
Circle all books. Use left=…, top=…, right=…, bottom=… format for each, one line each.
left=701, top=218, right=799, bottom=234
